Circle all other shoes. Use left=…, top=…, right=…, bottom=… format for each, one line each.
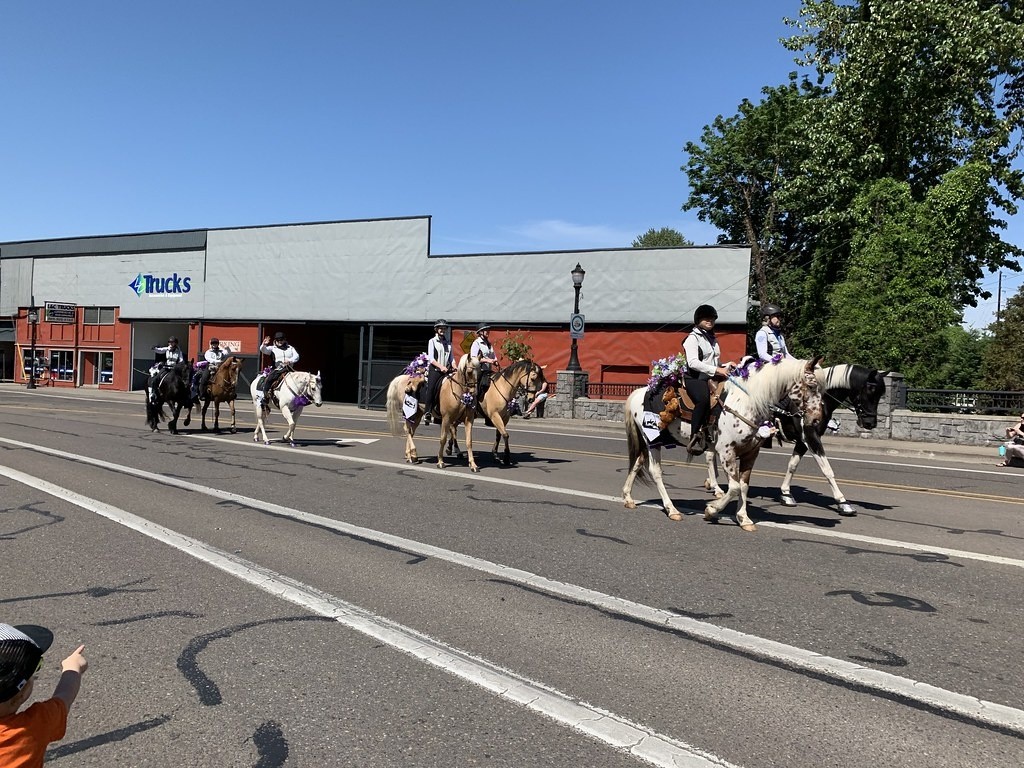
left=424, top=413, right=431, bottom=426
left=690, top=441, right=703, bottom=453
left=472, top=400, right=478, bottom=407
left=523, top=414, right=530, bottom=419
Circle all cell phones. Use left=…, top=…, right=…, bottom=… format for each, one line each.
left=1011, top=429, right=1014, bottom=431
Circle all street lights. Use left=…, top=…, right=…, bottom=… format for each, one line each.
left=566, top=263, right=585, bottom=370
left=27, top=308, right=38, bottom=388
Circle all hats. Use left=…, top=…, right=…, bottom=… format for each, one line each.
left=0, top=622, right=54, bottom=703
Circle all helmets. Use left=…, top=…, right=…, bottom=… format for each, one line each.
left=275, top=332, right=283, bottom=338
left=760, top=304, right=784, bottom=317
left=476, top=323, right=490, bottom=334
left=168, top=336, right=178, bottom=343
left=210, top=338, right=219, bottom=342
left=434, top=319, right=448, bottom=327
left=694, top=305, right=718, bottom=324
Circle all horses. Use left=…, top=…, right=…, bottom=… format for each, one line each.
left=387, top=357, right=548, bottom=473
left=250, top=367, right=323, bottom=446
left=145, top=355, right=246, bottom=434
left=621, top=353, right=893, bottom=531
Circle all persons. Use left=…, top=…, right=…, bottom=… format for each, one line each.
left=996, top=412, right=1024, bottom=468
left=682, top=304, right=737, bottom=455
left=424, top=318, right=458, bottom=419
left=469, top=323, right=497, bottom=401
left=151, top=336, right=184, bottom=397
left=198, top=338, right=226, bottom=401
left=0, top=623, right=87, bottom=768
left=259, top=331, right=300, bottom=406
left=753, top=304, right=797, bottom=363
